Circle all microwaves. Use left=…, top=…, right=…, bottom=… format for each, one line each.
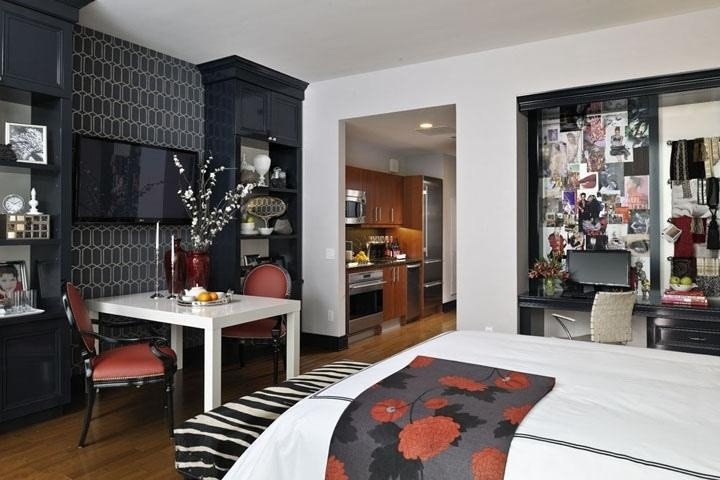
left=345, top=189, right=366, bottom=224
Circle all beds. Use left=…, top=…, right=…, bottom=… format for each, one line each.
left=222, top=331, right=720, bottom=479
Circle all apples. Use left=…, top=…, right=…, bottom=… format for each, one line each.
left=670, top=276, right=681, bottom=285
left=680, top=276, right=692, bottom=285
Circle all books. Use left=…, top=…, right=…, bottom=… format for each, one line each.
left=659, top=289, right=709, bottom=307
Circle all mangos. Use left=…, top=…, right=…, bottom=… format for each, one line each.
left=197, top=293, right=211, bottom=302
left=207, top=292, right=218, bottom=300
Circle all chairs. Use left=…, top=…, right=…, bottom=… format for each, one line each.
left=222, top=262, right=293, bottom=385
left=552, top=290, right=638, bottom=343
left=62, top=282, right=177, bottom=449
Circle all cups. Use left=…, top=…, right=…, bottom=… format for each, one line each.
left=346, top=251, right=354, bottom=257
left=4, top=288, right=37, bottom=314
left=215, top=292, right=224, bottom=299
left=241, top=222, right=255, bottom=232
left=182, top=296, right=195, bottom=303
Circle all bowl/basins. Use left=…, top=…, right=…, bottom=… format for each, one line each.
left=257, top=227, right=274, bottom=236
left=668, top=283, right=699, bottom=293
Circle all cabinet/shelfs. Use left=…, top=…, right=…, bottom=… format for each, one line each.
left=196, top=55, right=310, bottom=299
left=0, top=0, right=94, bottom=436
left=346, top=166, right=404, bottom=228
left=382, top=258, right=408, bottom=326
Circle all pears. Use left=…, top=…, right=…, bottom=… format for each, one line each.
left=354, top=251, right=369, bottom=261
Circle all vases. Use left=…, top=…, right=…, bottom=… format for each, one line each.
left=189, top=251, right=211, bottom=293
left=542, top=278, right=564, bottom=300
left=164, top=239, right=189, bottom=294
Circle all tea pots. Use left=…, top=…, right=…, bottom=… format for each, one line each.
left=183, top=283, right=207, bottom=300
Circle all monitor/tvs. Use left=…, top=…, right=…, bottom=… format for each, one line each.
left=72, top=132, right=198, bottom=226
left=566, top=249, right=632, bottom=294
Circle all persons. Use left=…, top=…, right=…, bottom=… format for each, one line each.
left=1, top=264, right=23, bottom=301
left=547, top=115, right=650, bottom=263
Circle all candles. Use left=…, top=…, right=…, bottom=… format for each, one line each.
left=171, top=234, right=175, bottom=267
left=155, top=221, right=160, bottom=250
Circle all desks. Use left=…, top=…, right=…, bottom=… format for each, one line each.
left=518, top=296, right=720, bottom=357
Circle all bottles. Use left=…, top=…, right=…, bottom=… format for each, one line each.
left=366, top=235, right=400, bottom=259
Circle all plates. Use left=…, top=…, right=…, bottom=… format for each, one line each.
left=0, top=303, right=46, bottom=320
left=240, top=230, right=260, bottom=235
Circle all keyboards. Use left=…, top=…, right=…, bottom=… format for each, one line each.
left=563, top=290, right=596, bottom=300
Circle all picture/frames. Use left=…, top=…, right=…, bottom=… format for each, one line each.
left=5, top=121, right=48, bottom=166
left=0, top=259, right=29, bottom=301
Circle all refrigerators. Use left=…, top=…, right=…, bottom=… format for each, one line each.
left=423, top=180, right=443, bottom=316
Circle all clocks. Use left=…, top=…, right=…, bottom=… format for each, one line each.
left=2, top=194, right=25, bottom=214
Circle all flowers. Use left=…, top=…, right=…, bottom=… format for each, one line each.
left=528, top=255, right=570, bottom=288
left=170, top=151, right=258, bottom=254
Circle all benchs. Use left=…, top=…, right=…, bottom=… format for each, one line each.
left=174, top=359, right=374, bottom=480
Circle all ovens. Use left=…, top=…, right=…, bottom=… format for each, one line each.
left=347, top=270, right=383, bottom=344
